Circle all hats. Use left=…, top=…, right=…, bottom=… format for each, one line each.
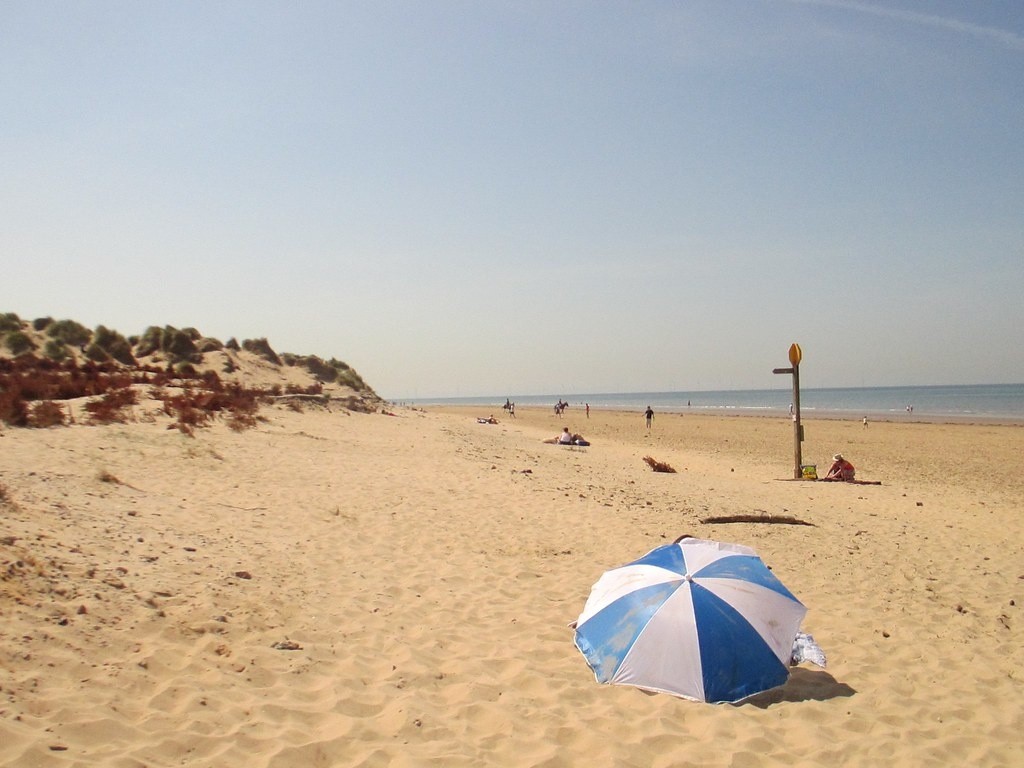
left=833, top=453, right=843, bottom=461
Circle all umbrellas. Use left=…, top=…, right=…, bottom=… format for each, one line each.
left=573, top=538, right=808, bottom=705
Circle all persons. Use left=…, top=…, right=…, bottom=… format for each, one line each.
left=789, top=402, right=793, bottom=415
left=558, top=427, right=573, bottom=444
left=559, top=399, right=561, bottom=405
left=863, top=416, right=868, bottom=428
left=824, top=453, right=856, bottom=482
left=489, top=415, right=498, bottom=424
left=907, top=405, right=913, bottom=412
left=585, top=404, right=590, bottom=419
left=507, top=399, right=510, bottom=406
left=509, top=403, right=515, bottom=418
left=642, top=406, right=654, bottom=429
left=381, top=410, right=395, bottom=416
